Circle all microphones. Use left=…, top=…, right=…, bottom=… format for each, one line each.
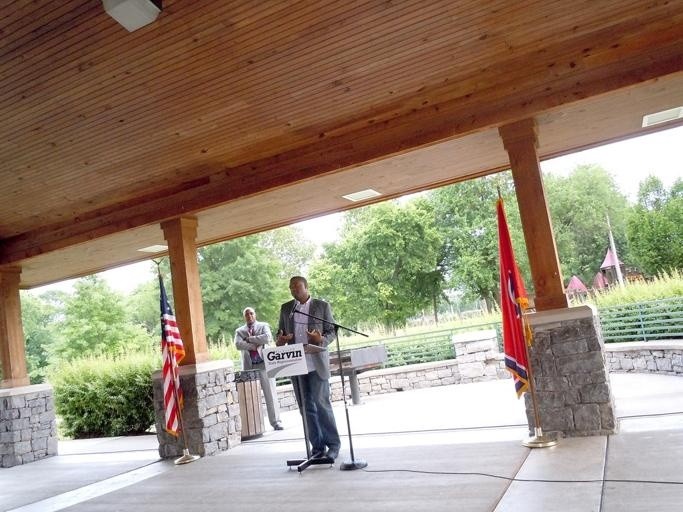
left=289, top=300, right=300, bottom=320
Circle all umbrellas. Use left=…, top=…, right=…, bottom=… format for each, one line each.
left=562, top=248, right=624, bottom=300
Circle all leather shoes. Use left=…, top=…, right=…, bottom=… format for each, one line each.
left=312, top=445, right=325, bottom=458
left=326, top=447, right=338, bottom=459
left=274, top=423, right=284, bottom=429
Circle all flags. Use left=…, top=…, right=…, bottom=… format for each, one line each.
left=495, top=197, right=535, bottom=400
left=158, top=274, right=187, bottom=441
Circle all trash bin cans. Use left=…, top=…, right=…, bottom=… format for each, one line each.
left=234, top=368, right=264, bottom=440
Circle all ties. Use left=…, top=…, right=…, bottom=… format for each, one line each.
left=249, top=327, right=257, bottom=359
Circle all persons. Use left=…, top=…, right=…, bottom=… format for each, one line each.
left=274, top=276, right=342, bottom=463
left=234, top=306, right=285, bottom=433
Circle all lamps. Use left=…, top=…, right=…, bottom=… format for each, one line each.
left=104, top=1, right=162, bottom=35
